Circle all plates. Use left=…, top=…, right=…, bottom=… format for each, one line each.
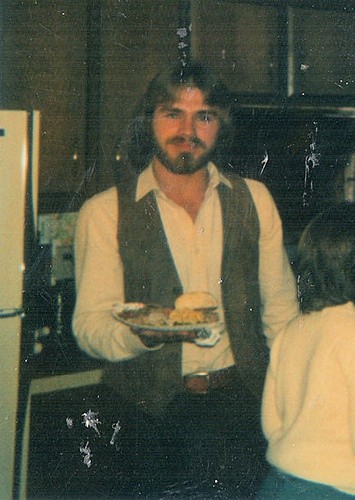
left=112, top=304, right=228, bottom=337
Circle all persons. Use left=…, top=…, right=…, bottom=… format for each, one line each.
left=256, top=203, right=355, bottom=500
left=72, top=63, right=300, bottom=500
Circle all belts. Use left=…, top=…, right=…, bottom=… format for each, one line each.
left=179, top=364, right=246, bottom=394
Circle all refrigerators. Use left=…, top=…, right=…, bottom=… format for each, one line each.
left=0, top=106, right=42, bottom=500
